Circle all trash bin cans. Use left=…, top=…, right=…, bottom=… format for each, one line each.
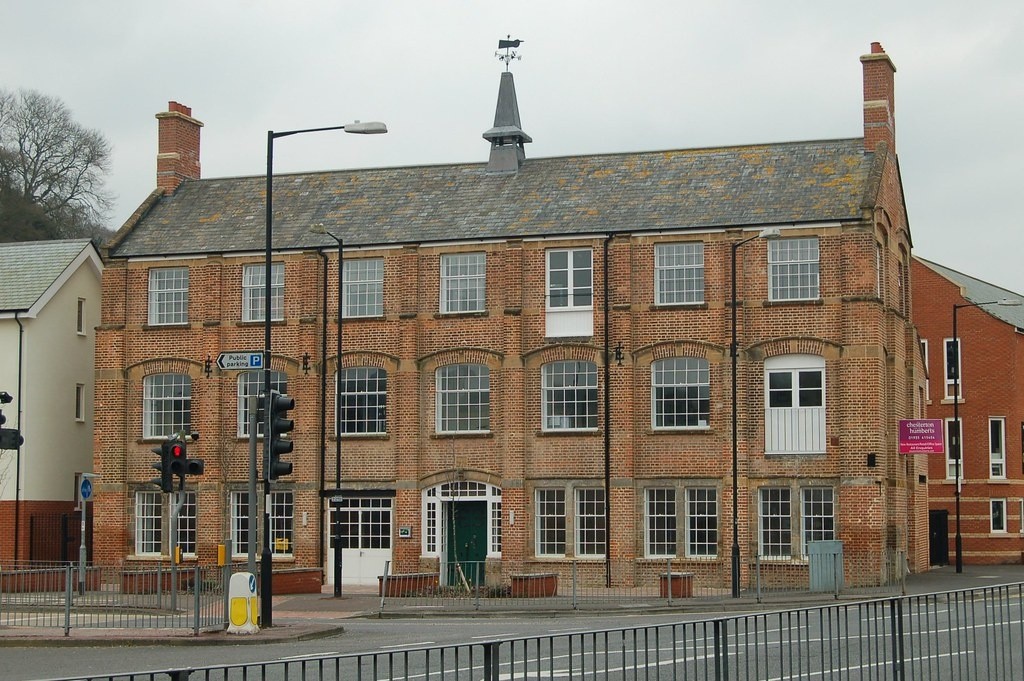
left=226, top=571, right=261, bottom=635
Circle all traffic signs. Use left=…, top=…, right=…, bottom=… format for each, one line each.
left=77, top=473, right=94, bottom=502
left=215, top=350, right=264, bottom=370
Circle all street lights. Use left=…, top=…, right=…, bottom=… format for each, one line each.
left=953, top=296, right=1023, bottom=573
left=259, top=123, right=388, bottom=630
left=603, top=232, right=617, bottom=588
left=731, top=227, right=781, bottom=599
left=309, top=223, right=343, bottom=597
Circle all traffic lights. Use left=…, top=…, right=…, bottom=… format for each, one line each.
left=150, top=439, right=174, bottom=494
left=169, top=440, right=204, bottom=475
left=268, top=388, right=295, bottom=484
